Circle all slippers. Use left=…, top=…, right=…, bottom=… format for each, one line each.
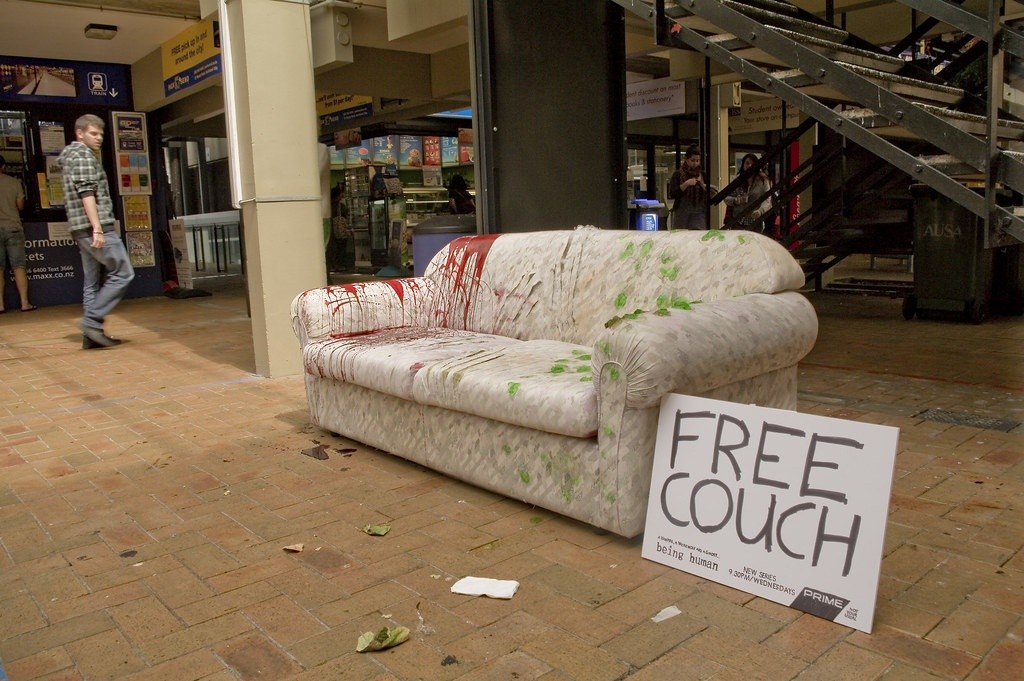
left=20, top=304, right=38, bottom=311
left=0, top=308, right=6, bottom=314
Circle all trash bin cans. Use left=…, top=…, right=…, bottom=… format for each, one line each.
left=905, top=169, right=988, bottom=318
left=410, top=211, right=476, bottom=277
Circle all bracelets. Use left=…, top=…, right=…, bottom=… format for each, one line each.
left=93, top=231, right=103, bottom=234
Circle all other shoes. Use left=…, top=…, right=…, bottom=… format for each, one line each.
left=81, top=326, right=121, bottom=349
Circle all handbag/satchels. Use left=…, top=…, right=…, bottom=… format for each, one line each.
left=723, top=202, right=748, bottom=228
left=332, top=204, right=351, bottom=238
left=698, top=180, right=719, bottom=200
left=460, top=202, right=475, bottom=214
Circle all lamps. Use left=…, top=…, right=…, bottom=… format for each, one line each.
left=84, top=23, right=118, bottom=40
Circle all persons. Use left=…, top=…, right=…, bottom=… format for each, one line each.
left=723, top=154, right=772, bottom=234
left=449, top=176, right=475, bottom=215
left=330, top=187, right=349, bottom=272
left=61, top=114, right=135, bottom=349
left=0, top=155, right=37, bottom=311
left=669, top=144, right=710, bottom=230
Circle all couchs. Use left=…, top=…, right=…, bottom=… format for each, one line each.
left=288, top=223, right=819, bottom=539
